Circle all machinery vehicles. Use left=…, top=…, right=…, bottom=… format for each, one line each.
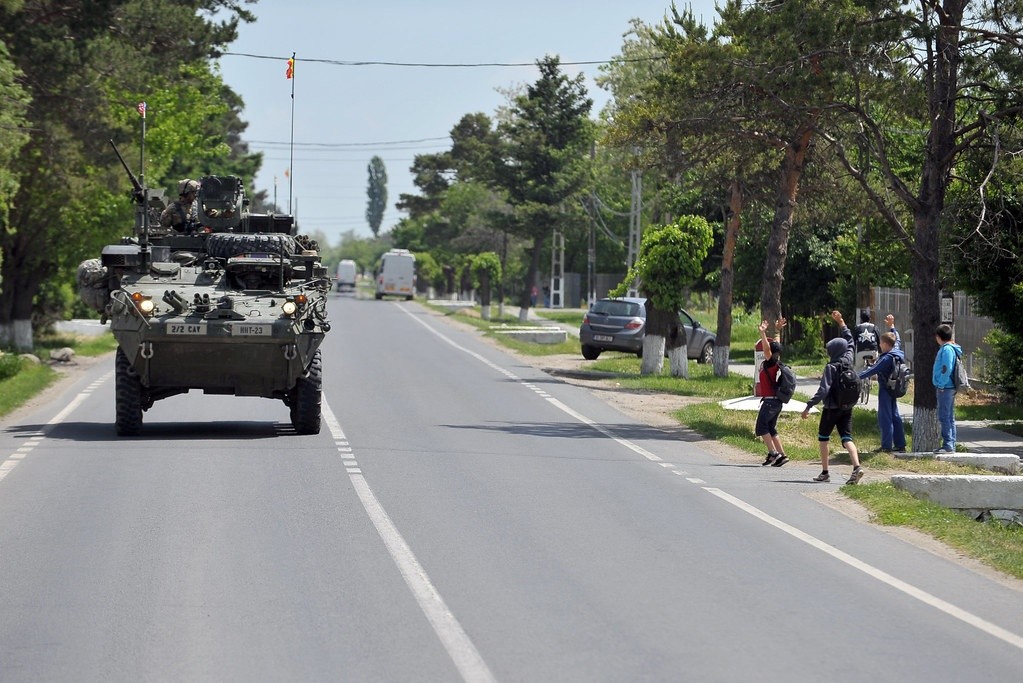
left=101, top=139, right=331, bottom=436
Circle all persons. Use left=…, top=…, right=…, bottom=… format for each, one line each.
left=858, top=314, right=907, bottom=453
left=801, top=311, right=864, bottom=485
left=852, top=309, right=882, bottom=386
left=160, top=179, right=206, bottom=233
left=754, top=318, right=789, bottom=467
left=932, top=324, right=962, bottom=454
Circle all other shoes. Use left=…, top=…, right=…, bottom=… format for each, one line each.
left=873, top=447, right=892, bottom=453
left=893, top=446, right=906, bottom=453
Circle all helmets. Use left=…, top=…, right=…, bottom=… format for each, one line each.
left=177, top=179, right=201, bottom=196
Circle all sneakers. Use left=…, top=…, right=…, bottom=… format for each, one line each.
left=933, top=447, right=954, bottom=453
left=845, top=468, right=864, bottom=486
left=772, top=456, right=789, bottom=467
left=812, top=474, right=830, bottom=482
left=762, top=451, right=782, bottom=467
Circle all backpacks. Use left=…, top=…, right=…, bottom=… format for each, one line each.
left=886, top=355, right=911, bottom=398
left=830, top=363, right=862, bottom=406
left=764, top=361, right=795, bottom=404
left=950, top=355, right=970, bottom=393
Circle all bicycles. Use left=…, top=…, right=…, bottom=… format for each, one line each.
left=855, top=356, right=877, bottom=404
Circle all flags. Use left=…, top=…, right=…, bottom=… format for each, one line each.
left=285, top=55, right=295, bottom=79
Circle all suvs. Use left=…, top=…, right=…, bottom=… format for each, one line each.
left=580, top=295, right=717, bottom=364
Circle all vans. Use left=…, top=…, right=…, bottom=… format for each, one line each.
left=375, top=248, right=417, bottom=300
left=337, top=259, right=357, bottom=289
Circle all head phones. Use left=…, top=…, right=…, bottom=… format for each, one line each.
left=182, top=180, right=191, bottom=197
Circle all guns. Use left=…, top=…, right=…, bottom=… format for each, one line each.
left=109, top=137, right=149, bottom=204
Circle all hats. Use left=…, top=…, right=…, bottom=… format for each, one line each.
left=860, top=309, right=871, bottom=321
